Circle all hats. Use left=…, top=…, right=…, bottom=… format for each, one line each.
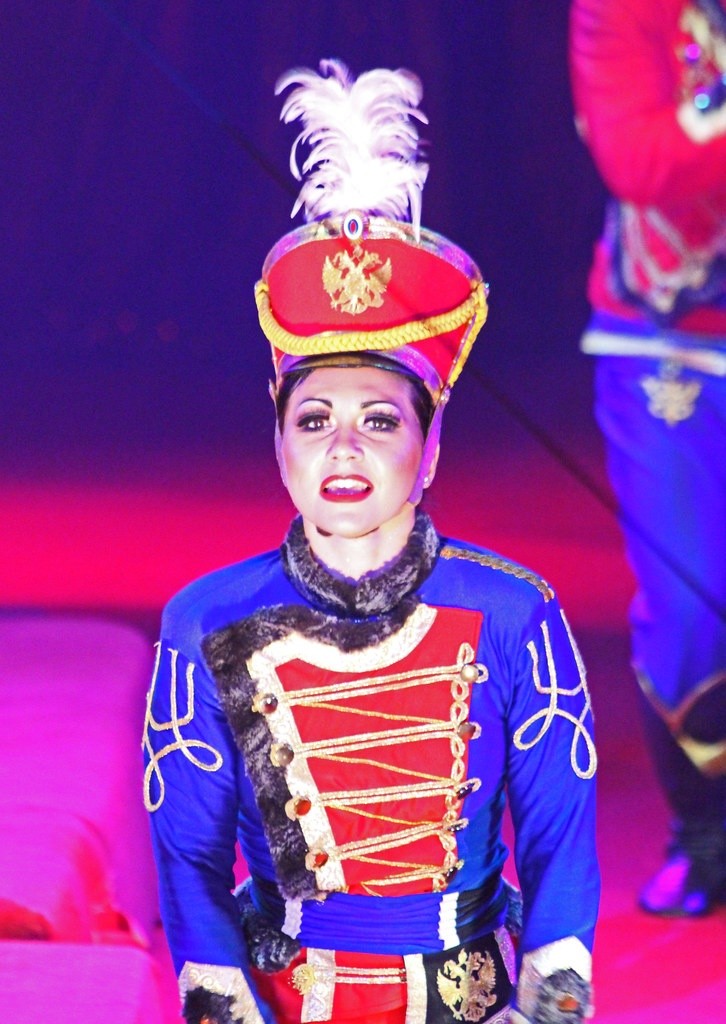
left=254, top=57, right=489, bottom=507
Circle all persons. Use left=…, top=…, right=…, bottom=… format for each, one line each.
left=135, top=325, right=603, bottom=1023
left=565, top=1, right=726, bottom=920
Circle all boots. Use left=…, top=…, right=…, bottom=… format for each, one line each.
left=638, top=669, right=726, bottom=915
